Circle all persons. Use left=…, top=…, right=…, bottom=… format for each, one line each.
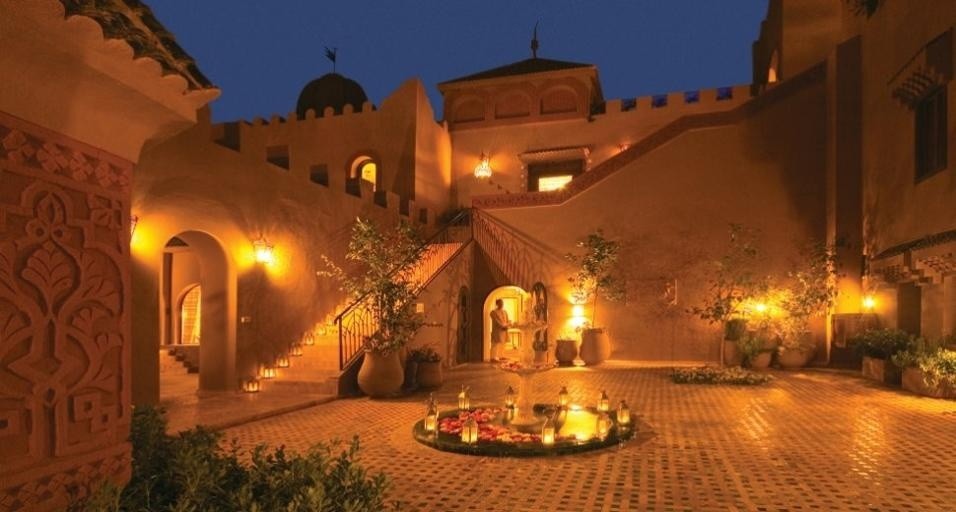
left=489, top=299, right=519, bottom=363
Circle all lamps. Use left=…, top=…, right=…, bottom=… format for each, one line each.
left=421, top=383, right=631, bottom=450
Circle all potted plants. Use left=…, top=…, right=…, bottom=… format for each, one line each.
left=845, top=324, right=956, bottom=401
left=318, top=213, right=446, bottom=395
left=554, top=226, right=628, bottom=367
left=689, top=218, right=857, bottom=374
